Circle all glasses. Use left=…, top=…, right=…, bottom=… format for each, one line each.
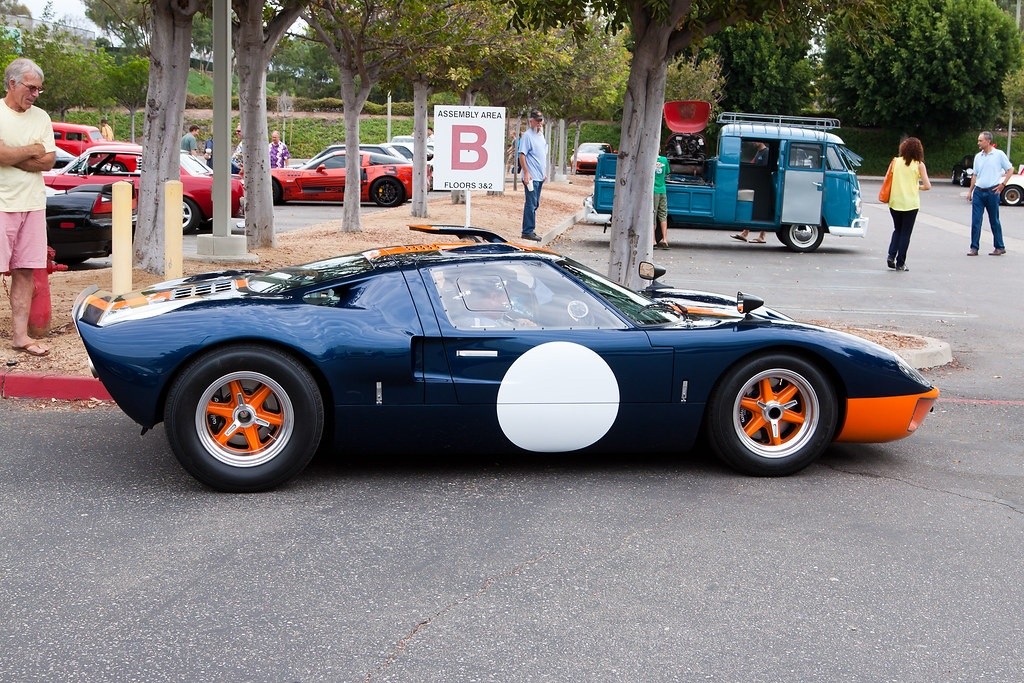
left=19, top=81, right=45, bottom=93
left=236, top=130, right=241, bottom=132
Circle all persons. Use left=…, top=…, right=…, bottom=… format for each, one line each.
left=230, top=123, right=246, bottom=175
left=965, top=130, right=1015, bottom=255
left=427, top=127, right=435, bottom=146
left=729, top=138, right=776, bottom=243
left=0, top=55, right=58, bottom=356
left=268, top=130, right=292, bottom=169
left=202, top=131, right=213, bottom=169
left=100, top=118, right=116, bottom=142
left=879, top=137, right=931, bottom=271
left=504, top=129, right=528, bottom=174
left=181, top=124, right=200, bottom=156
left=518, top=109, right=548, bottom=241
left=652, top=143, right=672, bottom=253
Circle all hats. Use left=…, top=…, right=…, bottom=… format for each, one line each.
left=530, top=110, right=544, bottom=121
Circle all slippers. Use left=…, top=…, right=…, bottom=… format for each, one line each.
left=730, top=235, right=747, bottom=242
left=660, top=242, right=670, bottom=249
left=12, top=340, right=50, bottom=356
left=749, top=238, right=766, bottom=243
left=653, top=247, right=658, bottom=249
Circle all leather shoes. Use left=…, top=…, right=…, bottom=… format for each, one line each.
left=989, top=248, right=1006, bottom=255
left=967, top=247, right=978, bottom=255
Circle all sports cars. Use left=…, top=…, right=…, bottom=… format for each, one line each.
left=259, top=151, right=428, bottom=210
left=75, top=223, right=935, bottom=491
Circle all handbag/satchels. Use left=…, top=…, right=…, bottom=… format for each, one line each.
left=878, top=157, right=896, bottom=203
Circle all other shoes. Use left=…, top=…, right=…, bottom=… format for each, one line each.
left=887, top=257, right=895, bottom=268
left=521, top=233, right=542, bottom=241
left=896, top=266, right=909, bottom=271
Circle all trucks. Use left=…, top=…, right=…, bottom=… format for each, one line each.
left=592, top=109, right=868, bottom=243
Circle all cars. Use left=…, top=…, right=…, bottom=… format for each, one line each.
left=33, top=124, right=252, bottom=256
left=993, top=169, right=1024, bottom=206
left=570, top=140, right=618, bottom=177
left=951, top=154, right=974, bottom=185
left=301, top=130, right=433, bottom=186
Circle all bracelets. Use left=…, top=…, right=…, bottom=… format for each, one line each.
left=1001, top=182, right=1006, bottom=187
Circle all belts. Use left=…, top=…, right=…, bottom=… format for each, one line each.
left=975, top=186, right=998, bottom=191
